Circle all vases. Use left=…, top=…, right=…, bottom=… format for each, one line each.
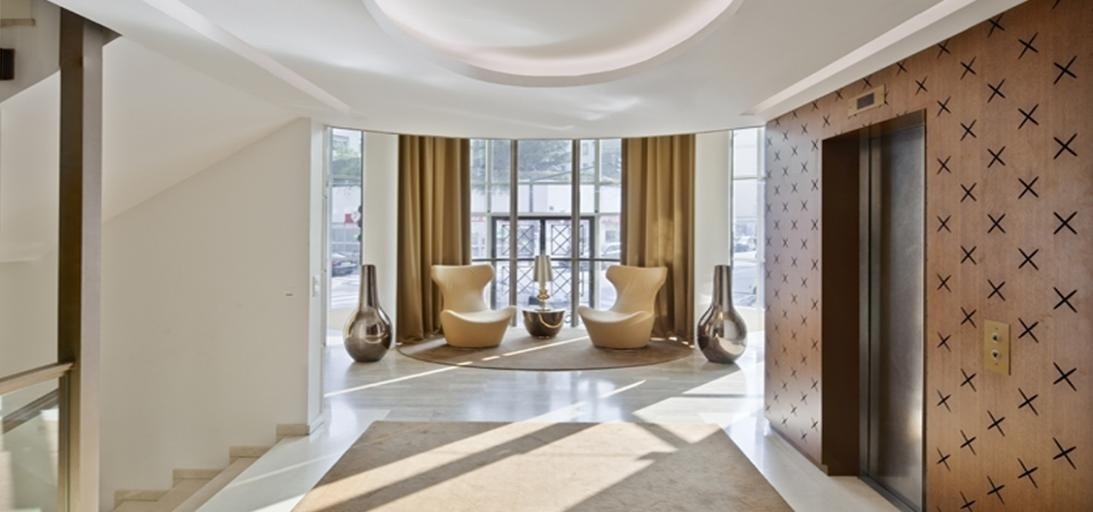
left=695, top=265, right=747, bottom=365
left=342, top=264, right=392, bottom=364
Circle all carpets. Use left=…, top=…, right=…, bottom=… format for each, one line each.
left=397, top=325, right=693, bottom=372
left=292, top=419, right=797, bottom=512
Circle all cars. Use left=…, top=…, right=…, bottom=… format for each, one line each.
left=558, top=229, right=620, bottom=271
left=331, top=252, right=357, bottom=276
left=733, top=235, right=758, bottom=295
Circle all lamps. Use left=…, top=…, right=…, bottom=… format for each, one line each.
left=532, top=254, right=555, bottom=311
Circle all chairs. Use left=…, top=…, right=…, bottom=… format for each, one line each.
left=428, top=260, right=517, bottom=350
left=576, top=264, right=670, bottom=349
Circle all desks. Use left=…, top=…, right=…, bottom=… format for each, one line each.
left=521, top=309, right=565, bottom=340
